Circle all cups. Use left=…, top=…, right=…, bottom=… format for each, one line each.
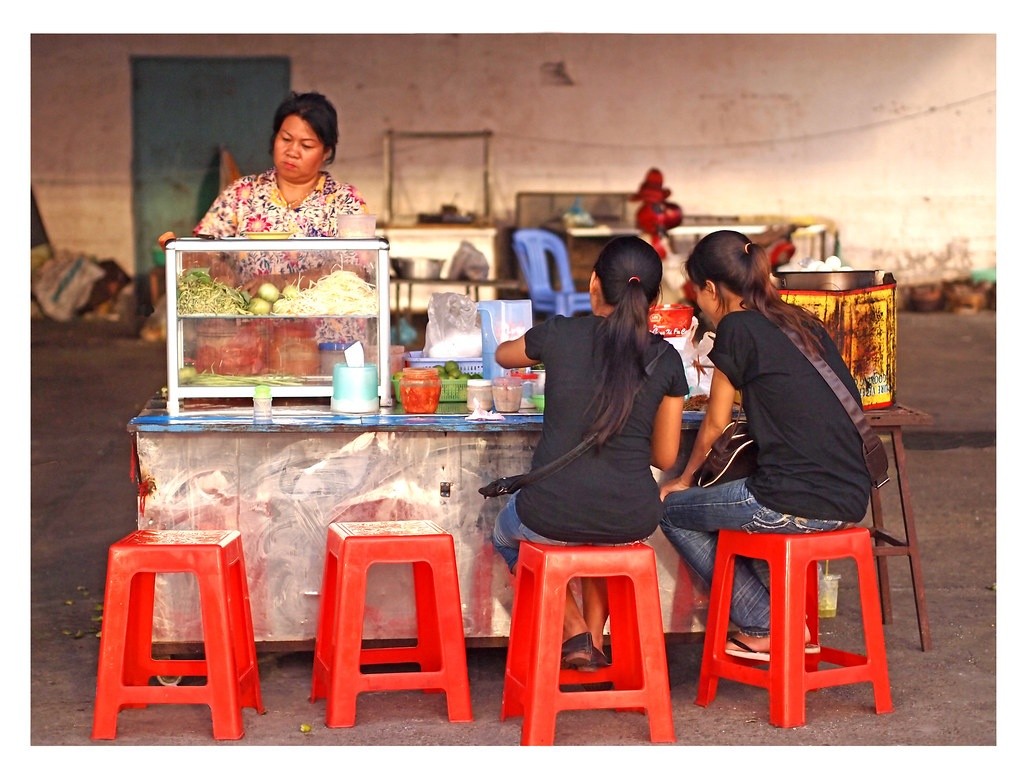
left=531, top=370, right=546, bottom=394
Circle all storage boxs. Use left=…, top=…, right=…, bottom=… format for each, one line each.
left=391, top=373, right=473, bottom=403
left=772, top=271, right=899, bottom=409
left=405, top=350, right=482, bottom=375
left=651, top=305, right=692, bottom=336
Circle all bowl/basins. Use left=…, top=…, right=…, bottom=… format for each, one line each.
left=336, top=213, right=377, bottom=237
left=647, top=303, right=694, bottom=337
left=391, top=257, right=446, bottom=279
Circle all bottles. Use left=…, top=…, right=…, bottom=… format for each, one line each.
left=252, top=386, right=272, bottom=424
left=571, top=197, right=582, bottom=214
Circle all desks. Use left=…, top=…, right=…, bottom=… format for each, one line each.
left=129, top=389, right=749, bottom=666
left=869, top=405, right=938, bottom=654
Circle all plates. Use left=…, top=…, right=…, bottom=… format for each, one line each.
left=242, top=232, right=294, bottom=239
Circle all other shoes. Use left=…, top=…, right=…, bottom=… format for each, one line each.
left=562, top=632, right=593, bottom=669
left=575, top=646, right=614, bottom=691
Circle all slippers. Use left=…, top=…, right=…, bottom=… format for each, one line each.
left=805, top=643, right=820, bottom=654
left=725, top=637, right=770, bottom=661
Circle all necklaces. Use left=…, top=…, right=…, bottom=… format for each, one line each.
left=287, top=189, right=313, bottom=210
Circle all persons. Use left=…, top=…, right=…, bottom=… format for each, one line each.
left=491, top=235, right=690, bottom=692
left=190, top=90, right=381, bottom=344
left=658, top=230, right=874, bottom=665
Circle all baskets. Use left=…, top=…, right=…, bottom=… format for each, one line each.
left=390, top=373, right=469, bottom=402
left=403, top=350, right=484, bottom=379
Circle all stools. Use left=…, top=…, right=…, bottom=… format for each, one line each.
left=93, top=529, right=264, bottom=742
left=695, top=527, right=894, bottom=728
left=500, top=540, right=677, bottom=746
left=313, top=521, right=473, bottom=728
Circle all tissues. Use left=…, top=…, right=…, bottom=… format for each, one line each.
left=330, top=341, right=380, bottom=414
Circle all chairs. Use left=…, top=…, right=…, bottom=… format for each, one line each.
left=515, top=230, right=591, bottom=315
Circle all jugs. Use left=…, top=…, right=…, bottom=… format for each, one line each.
left=478, top=300, right=532, bottom=413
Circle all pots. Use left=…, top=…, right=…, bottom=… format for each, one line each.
left=775, top=270, right=878, bottom=292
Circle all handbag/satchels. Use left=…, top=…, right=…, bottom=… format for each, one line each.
left=477, top=475, right=525, bottom=500
left=696, top=421, right=755, bottom=488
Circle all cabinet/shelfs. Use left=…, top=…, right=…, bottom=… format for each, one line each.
left=167, top=239, right=393, bottom=410
